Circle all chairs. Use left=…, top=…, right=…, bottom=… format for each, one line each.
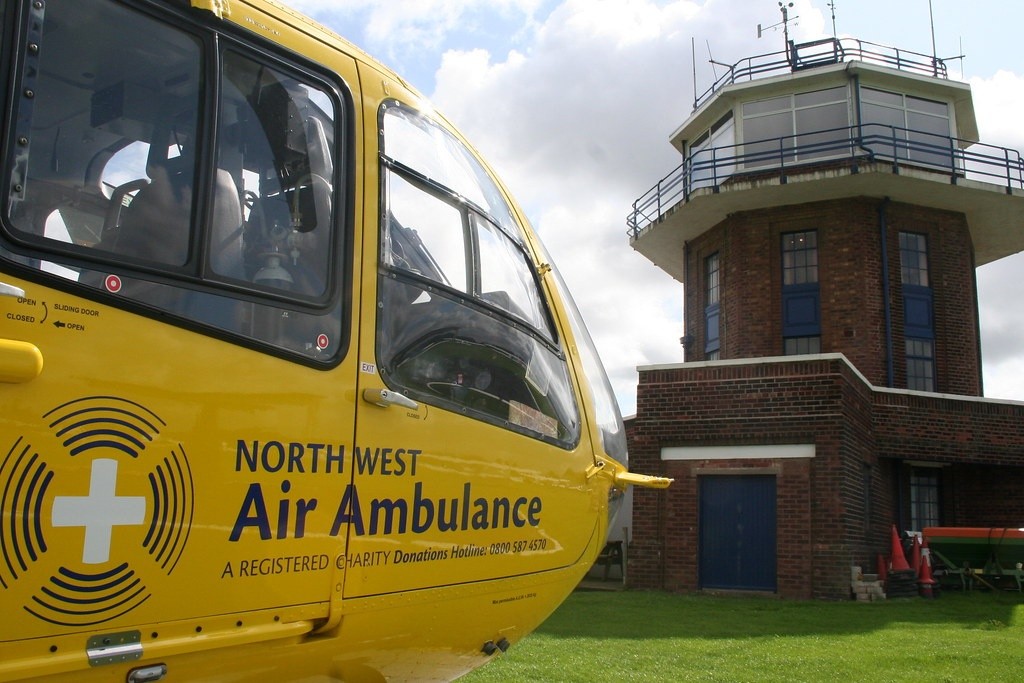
left=125, top=118, right=241, bottom=332
left=79, top=178, right=148, bottom=293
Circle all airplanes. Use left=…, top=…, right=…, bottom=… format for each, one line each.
left=0, top=1, right=678, bottom=682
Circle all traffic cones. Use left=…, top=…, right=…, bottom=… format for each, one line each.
left=891, top=523, right=937, bottom=597
left=878, top=556, right=890, bottom=583
